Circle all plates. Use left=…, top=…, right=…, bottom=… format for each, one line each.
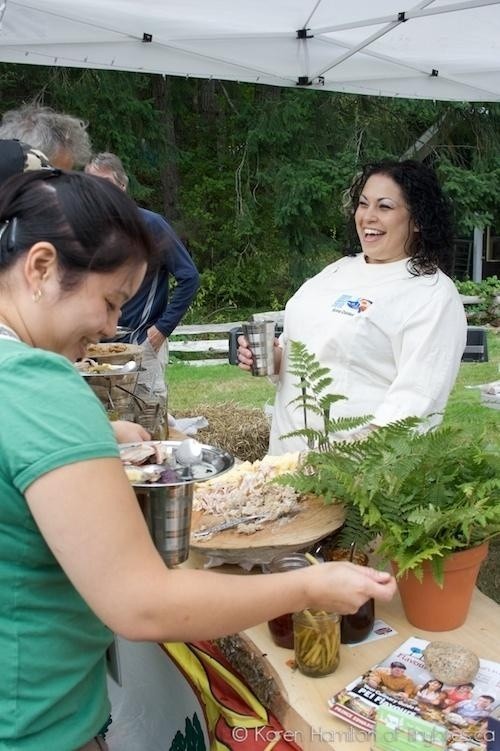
left=83, top=342, right=145, bottom=357
left=189, top=484, right=346, bottom=566
left=79, top=365, right=147, bottom=376
left=118, top=441, right=234, bottom=487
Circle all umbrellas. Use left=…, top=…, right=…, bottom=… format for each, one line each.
left=0, top=0, right=500, bottom=103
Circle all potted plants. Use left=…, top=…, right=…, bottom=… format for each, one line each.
left=262, top=338, right=500, bottom=632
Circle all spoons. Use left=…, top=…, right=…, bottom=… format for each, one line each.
left=310, top=546, right=321, bottom=565
left=349, top=542, right=355, bottom=563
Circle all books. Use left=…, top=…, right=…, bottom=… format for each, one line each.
left=326, top=634, right=500, bottom=750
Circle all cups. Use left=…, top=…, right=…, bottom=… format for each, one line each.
left=242, top=321, right=276, bottom=377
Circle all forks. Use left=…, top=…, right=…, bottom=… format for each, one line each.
left=195, top=496, right=294, bottom=537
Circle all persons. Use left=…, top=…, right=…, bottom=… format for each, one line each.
left=0, top=167, right=400, bottom=750
left=237, top=157, right=469, bottom=459
left=441, top=694, right=495, bottom=726
left=0, top=104, right=87, bottom=175
left=83, top=151, right=200, bottom=439
left=440, top=682, right=475, bottom=701
left=415, top=679, right=443, bottom=703
left=367, top=661, right=416, bottom=702
